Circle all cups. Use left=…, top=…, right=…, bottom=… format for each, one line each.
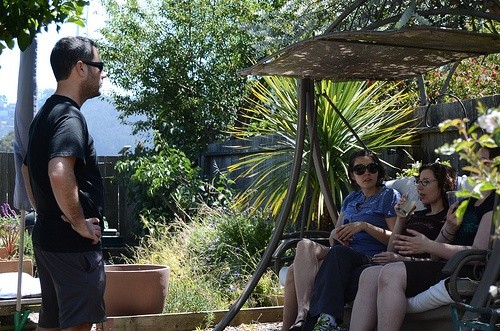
left=446, top=190, right=464, bottom=209
left=394, top=185, right=422, bottom=218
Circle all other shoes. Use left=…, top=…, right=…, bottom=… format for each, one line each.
left=312, top=313, right=342, bottom=331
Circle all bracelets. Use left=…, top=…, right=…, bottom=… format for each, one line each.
left=445, top=228, right=455, bottom=237
left=440, top=230, right=451, bottom=241
left=411, top=257, right=415, bottom=261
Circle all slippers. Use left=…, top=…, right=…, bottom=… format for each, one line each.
left=288, top=307, right=310, bottom=331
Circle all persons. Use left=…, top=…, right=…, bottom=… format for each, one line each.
left=280, top=145, right=500, bottom=331
left=21, top=36, right=108, bottom=331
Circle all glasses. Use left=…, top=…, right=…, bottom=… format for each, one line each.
left=351, top=162, right=380, bottom=175
left=414, top=180, right=438, bottom=186
left=83, top=61, right=104, bottom=73
